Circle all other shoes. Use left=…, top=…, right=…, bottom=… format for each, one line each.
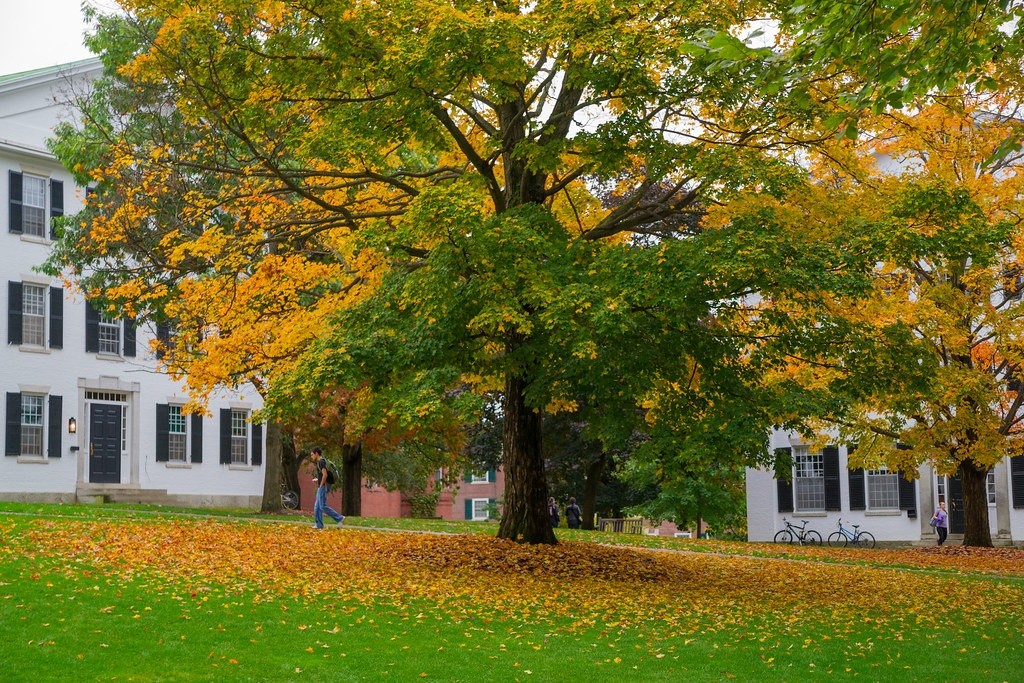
left=312, top=526, right=319, bottom=529
left=336, top=516, right=345, bottom=529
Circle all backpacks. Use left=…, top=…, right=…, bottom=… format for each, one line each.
left=317, top=458, right=339, bottom=485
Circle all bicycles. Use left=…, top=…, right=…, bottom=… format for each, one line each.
left=773, top=516, right=822, bottom=547
left=281, top=484, right=299, bottom=509
left=827, top=518, right=876, bottom=550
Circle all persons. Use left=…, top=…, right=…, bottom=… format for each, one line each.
left=933, top=502, right=948, bottom=546
left=311, top=446, right=345, bottom=528
left=565, top=497, right=583, bottom=529
left=548, top=497, right=560, bottom=528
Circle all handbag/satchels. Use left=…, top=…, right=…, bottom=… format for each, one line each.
left=928, top=516, right=937, bottom=527
left=578, top=518, right=583, bottom=524
